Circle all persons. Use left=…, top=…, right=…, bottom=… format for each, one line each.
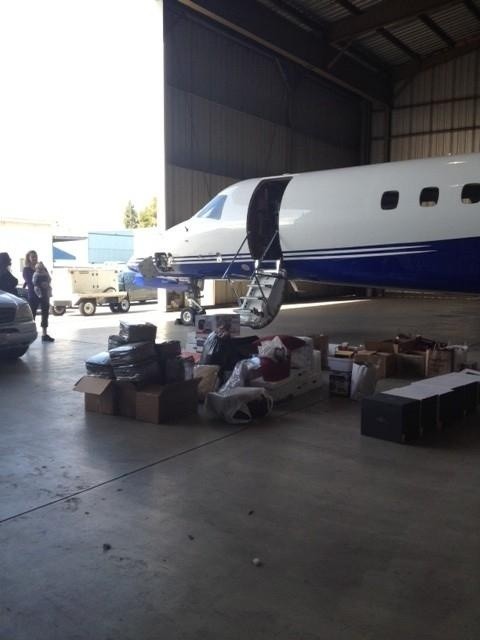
left=23, top=251, right=54, bottom=341
left=0, top=250, right=18, bottom=295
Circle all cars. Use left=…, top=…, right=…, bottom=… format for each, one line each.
left=0, top=287, right=39, bottom=363
left=115, top=258, right=204, bottom=301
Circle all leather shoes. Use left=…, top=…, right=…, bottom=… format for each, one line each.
left=42, top=335, right=55, bottom=341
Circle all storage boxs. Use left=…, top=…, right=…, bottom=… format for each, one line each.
left=73, top=315, right=480, bottom=445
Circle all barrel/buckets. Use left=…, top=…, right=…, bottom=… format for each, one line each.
left=453, top=350, right=479, bottom=373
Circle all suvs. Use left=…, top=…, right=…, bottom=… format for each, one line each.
left=44, top=267, right=131, bottom=314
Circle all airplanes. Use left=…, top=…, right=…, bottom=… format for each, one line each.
left=129, top=152, right=480, bottom=335
